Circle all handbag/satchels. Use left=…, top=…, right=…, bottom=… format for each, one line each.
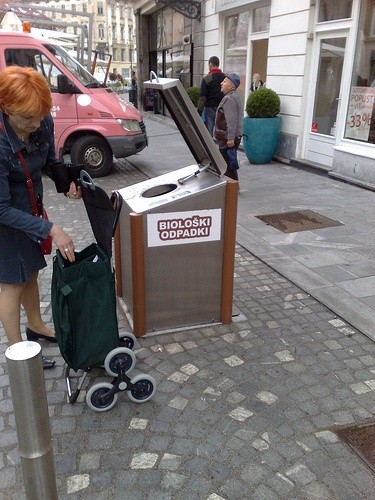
left=39, top=207, right=52, bottom=255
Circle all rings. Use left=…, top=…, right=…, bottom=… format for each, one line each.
left=73, top=192, right=77, bottom=195
left=64, top=248, right=68, bottom=251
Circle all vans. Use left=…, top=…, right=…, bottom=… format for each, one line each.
left=0, top=20, right=149, bottom=177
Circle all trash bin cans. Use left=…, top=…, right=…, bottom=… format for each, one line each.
left=110, top=70, right=239, bottom=339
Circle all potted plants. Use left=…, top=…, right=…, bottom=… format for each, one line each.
left=242, top=87, right=282, bottom=165
left=186, top=87, right=206, bottom=123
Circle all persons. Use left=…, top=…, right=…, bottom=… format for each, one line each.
left=249, top=73, right=263, bottom=94
left=213, top=73, right=243, bottom=194
left=0, top=64, right=82, bottom=370
left=196, top=56, right=225, bottom=137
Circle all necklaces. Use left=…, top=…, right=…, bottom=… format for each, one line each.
left=18, top=133, right=25, bottom=142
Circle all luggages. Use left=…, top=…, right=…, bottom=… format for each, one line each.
left=51, top=168, right=157, bottom=413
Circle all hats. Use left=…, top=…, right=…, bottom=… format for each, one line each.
left=224, top=73, right=240, bottom=88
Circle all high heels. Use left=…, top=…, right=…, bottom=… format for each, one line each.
left=42, top=355, right=55, bottom=368
left=26, top=323, right=57, bottom=343
